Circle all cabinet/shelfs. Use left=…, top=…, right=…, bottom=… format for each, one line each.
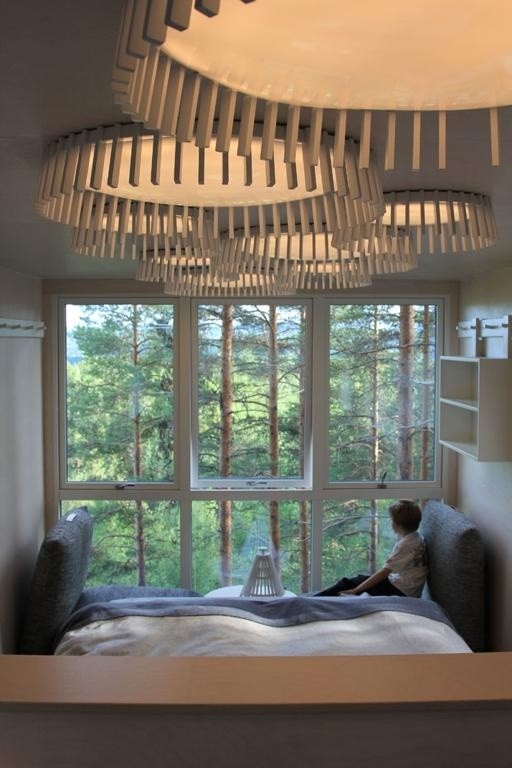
left=439, top=315, right=512, bottom=461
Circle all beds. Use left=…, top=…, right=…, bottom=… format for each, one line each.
left=55, top=596, right=473, bottom=656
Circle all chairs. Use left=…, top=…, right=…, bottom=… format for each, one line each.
left=361, top=500, right=484, bottom=651
left=22, top=507, right=196, bottom=655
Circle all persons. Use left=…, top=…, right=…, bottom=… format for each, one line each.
left=315, top=499, right=426, bottom=597
